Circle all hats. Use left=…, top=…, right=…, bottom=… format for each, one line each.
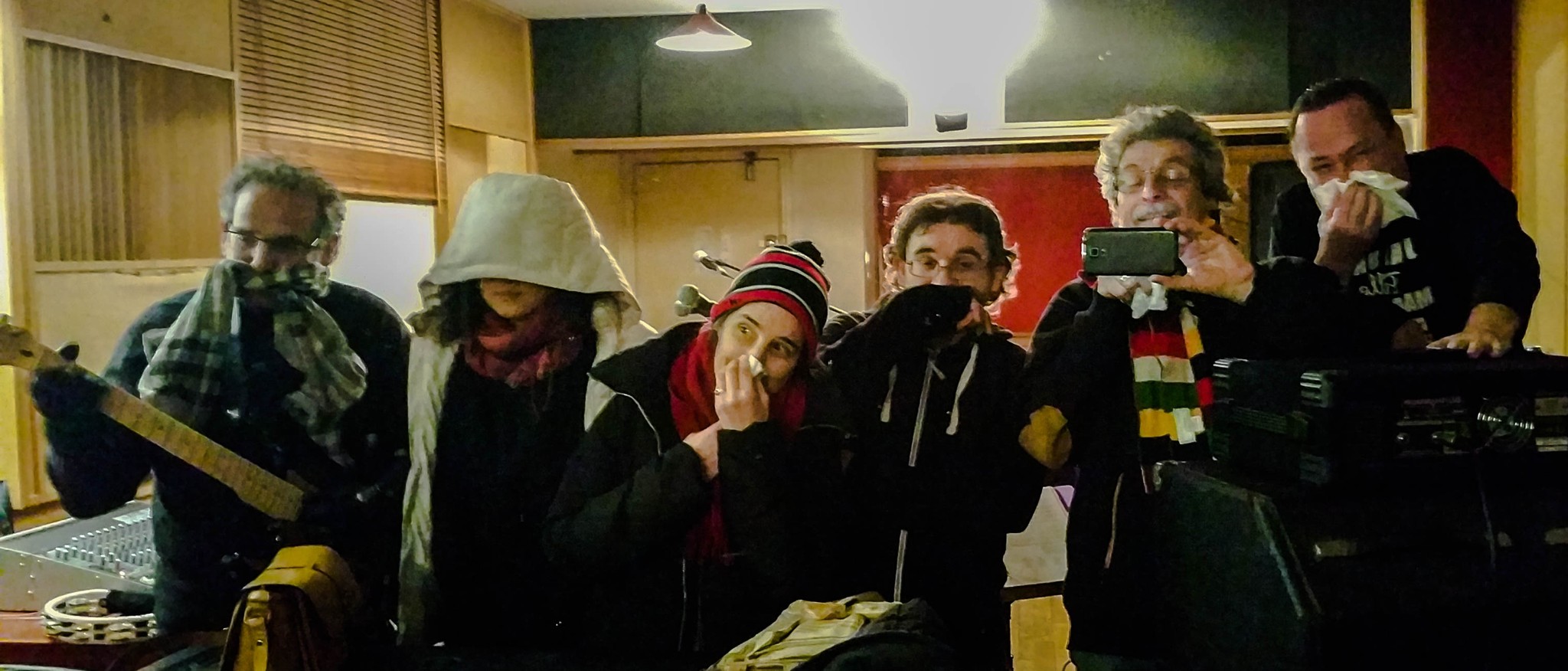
left=709, top=241, right=830, bottom=368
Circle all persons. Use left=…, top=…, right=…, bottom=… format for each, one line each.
left=399, top=174, right=660, bottom=671
left=33, top=153, right=410, bottom=671
left=1033, top=103, right=1431, bottom=671
left=546, top=243, right=857, bottom=671
left=816, top=184, right=1048, bottom=671
left=1267, top=78, right=1541, bottom=357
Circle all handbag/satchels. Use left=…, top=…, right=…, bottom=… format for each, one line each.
left=218, top=545, right=363, bottom=671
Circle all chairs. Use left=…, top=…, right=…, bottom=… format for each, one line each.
left=1160, top=464, right=1322, bottom=671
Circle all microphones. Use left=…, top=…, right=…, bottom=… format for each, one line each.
left=693, top=250, right=728, bottom=276
left=675, top=284, right=715, bottom=320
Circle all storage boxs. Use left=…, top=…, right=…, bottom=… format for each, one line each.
left=1211, top=349, right=1568, bottom=498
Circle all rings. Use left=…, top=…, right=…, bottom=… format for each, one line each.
left=1119, top=276, right=1131, bottom=283
left=714, top=389, right=726, bottom=395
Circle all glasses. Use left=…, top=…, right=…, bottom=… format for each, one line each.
left=226, top=227, right=316, bottom=257
left=905, top=256, right=992, bottom=278
left=1113, top=167, right=1200, bottom=193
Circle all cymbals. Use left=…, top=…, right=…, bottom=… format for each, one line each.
left=39, top=588, right=161, bottom=644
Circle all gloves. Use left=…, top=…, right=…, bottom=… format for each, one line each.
left=32, top=342, right=107, bottom=434
left=298, top=484, right=380, bottom=546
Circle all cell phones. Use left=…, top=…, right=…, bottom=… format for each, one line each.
left=1081, top=226, right=1180, bottom=278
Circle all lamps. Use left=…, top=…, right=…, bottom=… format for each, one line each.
left=935, top=112, right=967, bottom=132
left=655, top=3, right=752, bottom=52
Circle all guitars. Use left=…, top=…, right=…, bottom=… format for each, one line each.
left=0, top=311, right=401, bottom=622
